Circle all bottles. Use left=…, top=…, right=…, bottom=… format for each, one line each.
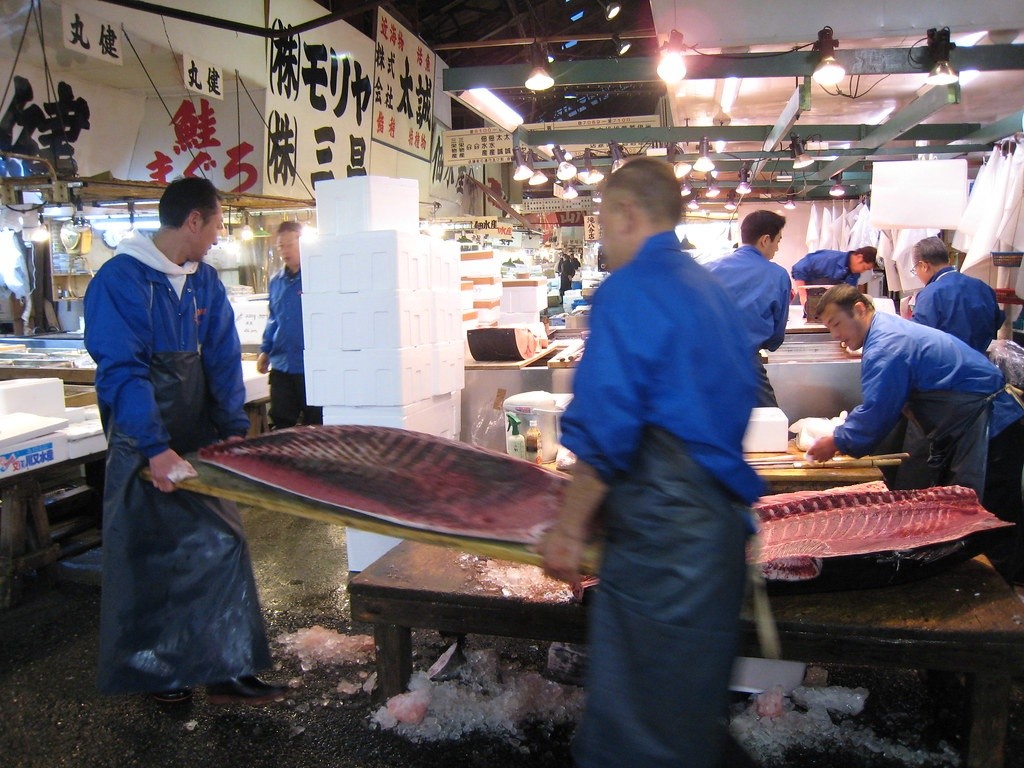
left=525, top=421, right=542, bottom=465
left=57, top=284, right=69, bottom=298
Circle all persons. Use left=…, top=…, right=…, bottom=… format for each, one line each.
left=558, top=247, right=583, bottom=304
left=698, top=210, right=792, bottom=408
left=908, top=235, right=1000, bottom=355
left=539, top=155, right=768, bottom=768
left=257, top=219, right=325, bottom=429
left=804, top=282, right=1024, bottom=588
left=792, top=246, right=877, bottom=307
left=83, top=173, right=285, bottom=707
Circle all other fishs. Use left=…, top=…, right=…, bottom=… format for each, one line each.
left=140, top=420, right=1019, bottom=585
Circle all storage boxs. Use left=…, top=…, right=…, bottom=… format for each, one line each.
left=742, top=407, right=788, bottom=454
left=1, top=175, right=563, bottom=572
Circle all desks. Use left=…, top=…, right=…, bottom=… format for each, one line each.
left=347, top=538, right=1024, bottom=768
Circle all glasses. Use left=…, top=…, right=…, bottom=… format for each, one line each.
left=909, top=262, right=919, bottom=276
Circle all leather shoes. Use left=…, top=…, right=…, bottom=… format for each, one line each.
left=144, top=671, right=195, bottom=703
left=206, top=675, right=294, bottom=706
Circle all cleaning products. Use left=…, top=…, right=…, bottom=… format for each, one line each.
left=504, top=413, right=526, bottom=459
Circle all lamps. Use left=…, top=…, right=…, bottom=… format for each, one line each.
left=500, top=132, right=844, bottom=212
left=598, top=0, right=622, bottom=22
left=810, top=27, right=846, bottom=87
left=916, top=26, right=958, bottom=85
left=654, top=27, right=697, bottom=81
left=525, top=41, right=555, bottom=92
left=612, top=34, right=632, bottom=58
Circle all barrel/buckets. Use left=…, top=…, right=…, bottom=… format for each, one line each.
left=57, top=300, right=80, bottom=331
